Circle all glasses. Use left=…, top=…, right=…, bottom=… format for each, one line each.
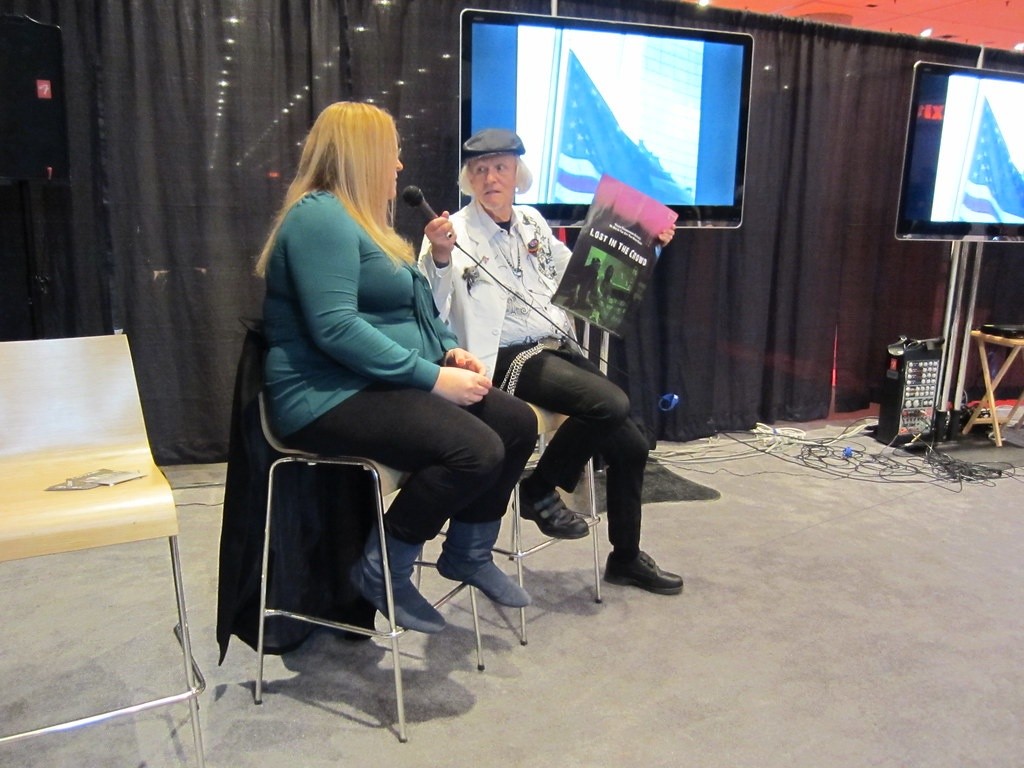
left=398, top=148, right=401, bottom=154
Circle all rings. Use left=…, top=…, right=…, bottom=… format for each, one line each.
left=447, top=232, right=452, bottom=239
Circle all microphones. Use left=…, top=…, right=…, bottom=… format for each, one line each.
left=402, top=186, right=460, bottom=248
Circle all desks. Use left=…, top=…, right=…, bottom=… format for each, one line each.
left=962, top=330, right=1024, bottom=447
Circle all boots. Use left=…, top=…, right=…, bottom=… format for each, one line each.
left=349, top=525, right=446, bottom=633
left=437, top=520, right=531, bottom=607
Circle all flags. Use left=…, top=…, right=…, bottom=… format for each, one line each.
left=959, top=99, right=1024, bottom=223
left=550, top=49, right=684, bottom=203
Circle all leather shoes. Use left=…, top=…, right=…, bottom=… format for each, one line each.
left=512, top=478, right=590, bottom=539
left=604, top=551, right=684, bottom=595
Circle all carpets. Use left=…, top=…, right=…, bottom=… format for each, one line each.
left=858, top=424, right=1024, bottom=474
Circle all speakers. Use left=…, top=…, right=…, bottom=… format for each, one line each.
left=878, top=342, right=942, bottom=446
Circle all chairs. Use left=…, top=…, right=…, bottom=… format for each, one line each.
left=415, top=401, right=601, bottom=644
left=0, top=334, right=206, bottom=768
left=255, top=390, right=486, bottom=743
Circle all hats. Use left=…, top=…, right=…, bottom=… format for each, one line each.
left=463, top=128, right=525, bottom=158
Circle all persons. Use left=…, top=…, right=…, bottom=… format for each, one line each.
left=416, top=129, right=683, bottom=594
left=575, top=259, right=614, bottom=308
left=255, top=100, right=538, bottom=633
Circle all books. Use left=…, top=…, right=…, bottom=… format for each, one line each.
left=551, top=174, right=679, bottom=337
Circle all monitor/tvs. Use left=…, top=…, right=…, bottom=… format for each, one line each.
left=459, top=8, right=754, bottom=228
left=895, top=60, right=1024, bottom=244
